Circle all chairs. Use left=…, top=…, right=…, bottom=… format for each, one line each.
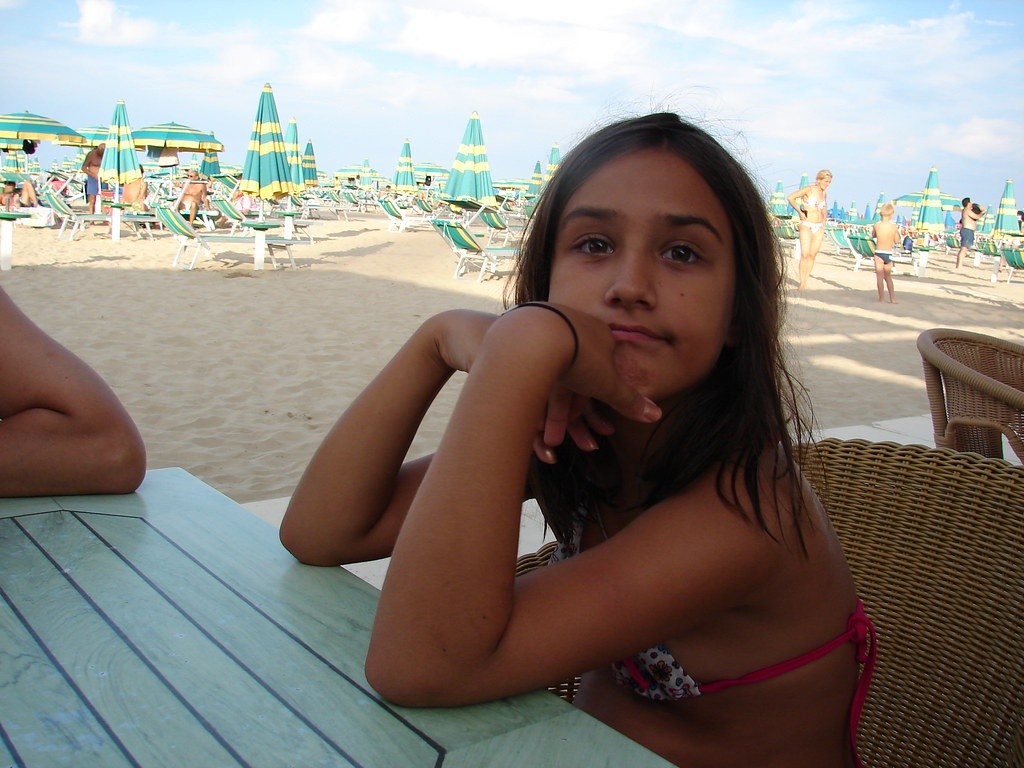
left=0, top=154, right=1024, bottom=284
left=511, top=437, right=1024, bottom=768
left=916, top=328, right=1024, bottom=467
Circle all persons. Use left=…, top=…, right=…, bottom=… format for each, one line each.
left=121, top=164, right=155, bottom=228
left=335, top=177, right=342, bottom=189
left=0, top=288, right=147, bottom=497
left=356, top=175, right=361, bottom=185
left=350, top=178, right=356, bottom=185
left=957, top=198, right=987, bottom=268
left=378, top=185, right=394, bottom=200
left=81, top=143, right=109, bottom=226
left=279, top=116, right=878, bottom=768
left=348, top=177, right=351, bottom=183
left=787, top=169, right=831, bottom=291
left=1004, top=211, right=1024, bottom=249
left=216, top=192, right=252, bottom=226
left=417, top=189, right=440, bottom=207
left=0, top=180, right=42, bottom=208
left=872, top=204, right=900, bottom=303
left=174, top=169, right=209, bottom=225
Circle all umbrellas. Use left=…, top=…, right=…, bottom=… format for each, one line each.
left=767, top=166, right=1024, bottom=282
left=333, top=109, right=562, bottom=212
left=0, top=82, right=325, bottom=242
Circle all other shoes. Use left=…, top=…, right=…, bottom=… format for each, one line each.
left=139, top=222, right=155, bottom=229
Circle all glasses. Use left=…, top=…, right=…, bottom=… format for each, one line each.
left=187, top=174, right=196, bottom=178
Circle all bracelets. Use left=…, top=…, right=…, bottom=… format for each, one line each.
left=510, top=302, right=580, bottom=366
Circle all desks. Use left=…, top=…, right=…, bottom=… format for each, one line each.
left=0, top=465, right=678, bottom=768
left=0, top=212, right=31, bottom=271
left=275, top=211, right=303, bottom=240
left=983, top=251, right=1003, bottom=284
left=240, top=221, right=281, bottom=270
left=102, top=203, right=132, bottom=242
left=971, top=248, right=986, bottom=267
left=913, top=247, right=936, bottom=273
left=791, top=234, right=802, bottom=260
